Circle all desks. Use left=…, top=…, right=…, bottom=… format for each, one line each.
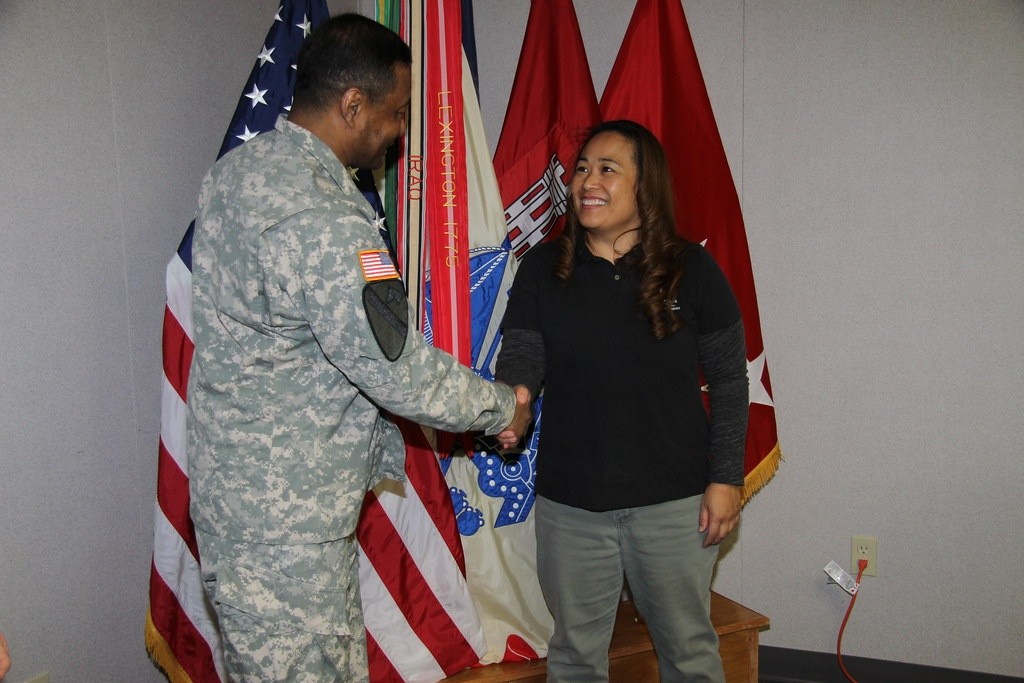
left=437, top=590, right=771, bottom=683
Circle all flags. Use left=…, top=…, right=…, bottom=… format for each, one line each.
left=141, top=0, right=783, bottom=683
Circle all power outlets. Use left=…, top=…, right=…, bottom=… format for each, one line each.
left=851, top=534, right=878, bottom=577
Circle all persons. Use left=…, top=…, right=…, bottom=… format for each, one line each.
left=181, top=10, right=531, bottom=683
left=492, top=122, right=748, bottom=682
